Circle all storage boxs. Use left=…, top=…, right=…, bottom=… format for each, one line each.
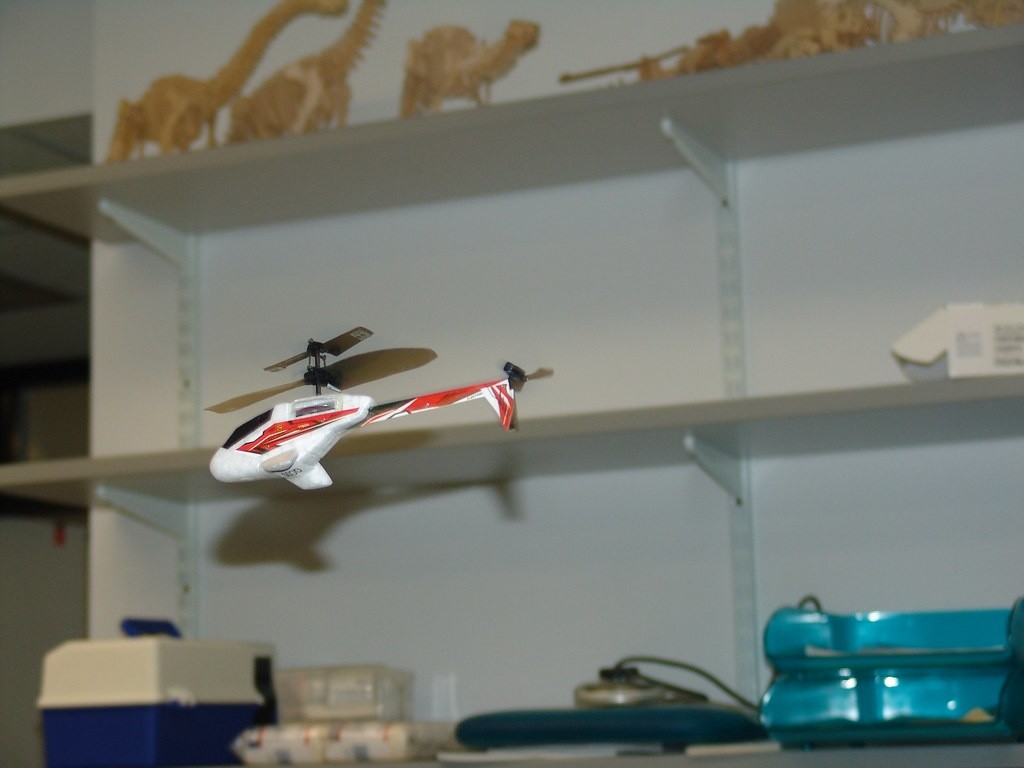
left=35, top=607, right=448, bottom=766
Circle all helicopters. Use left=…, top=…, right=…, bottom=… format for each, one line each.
left=204, top=327, right=552, bottom=491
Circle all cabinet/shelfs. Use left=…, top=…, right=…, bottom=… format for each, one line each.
left=1, top=19, right=1023, bottom=507
